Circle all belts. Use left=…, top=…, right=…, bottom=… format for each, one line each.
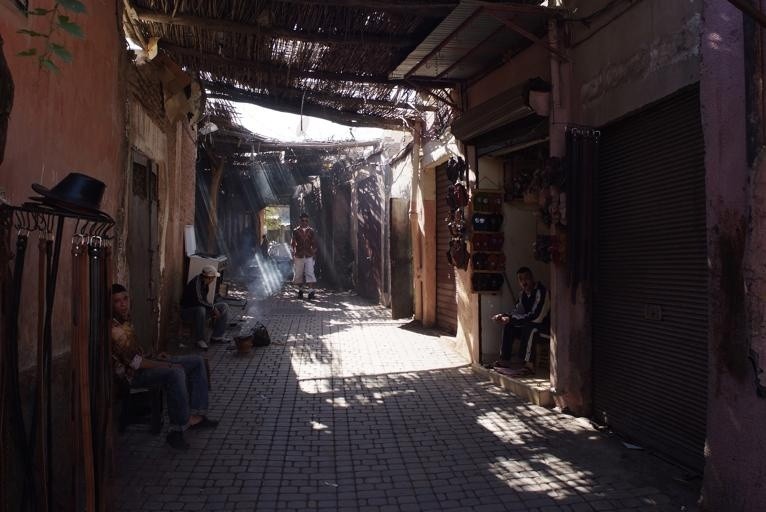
left=565, top=128, right=601, bottom=310
left=9, top=228, right=113, bottom=512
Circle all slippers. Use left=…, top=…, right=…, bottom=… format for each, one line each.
left=447, top=154, right=466, bottom=265
left=191, top=415, right=219, bottom=428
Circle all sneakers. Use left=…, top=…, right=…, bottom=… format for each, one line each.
left=198, top=339, right=208, bottom=349
left=210, top=338, right=231, bottom=344
left=483, top=361, right=499, bottom=368
left=298, top=290, right=303, bottom=299
left=308, top=291, right=314, bottom=299
left=510, top=367, right=534, bottom=375
left=471, top=191, right=506, bottom=291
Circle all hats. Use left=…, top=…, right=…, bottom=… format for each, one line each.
left=25, top=172, right=105, bottom=217
left=202, top=266, right=221, bottom=277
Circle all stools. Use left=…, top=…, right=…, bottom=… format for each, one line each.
left=118, top=389, right=165, bottom=435
left=175, top=314, right=213, bottom=349
left=535, top=332, right=549, bottom=369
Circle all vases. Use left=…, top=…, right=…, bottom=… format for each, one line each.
left=234, top=335, right=253, bottom=352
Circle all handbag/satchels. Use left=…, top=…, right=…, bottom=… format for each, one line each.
left=253, top=321, right=270, bottom=347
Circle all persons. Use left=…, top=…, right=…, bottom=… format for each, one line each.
left=490, top=267, right=551, bottom=376
left=180, top=265, right=231, bottom=349
left=261, top=234, right=268, bottom=260
left=290, top=213, right=319, bottom=299
left=109, top=283, right=219, bottom=448
